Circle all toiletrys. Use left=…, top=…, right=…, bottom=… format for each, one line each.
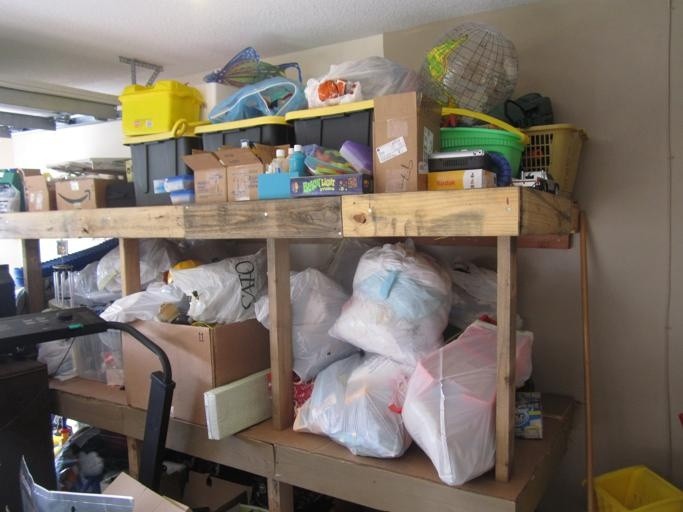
left=264, top=144, right=305, bottom=174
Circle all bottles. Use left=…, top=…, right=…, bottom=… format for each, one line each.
left=267, top=145, right=307, bottom=178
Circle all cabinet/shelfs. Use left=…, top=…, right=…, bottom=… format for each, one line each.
left=0, top=186, right=578, bottom=512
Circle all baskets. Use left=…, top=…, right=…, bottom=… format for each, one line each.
left=439, top=107, right=588, bottom=193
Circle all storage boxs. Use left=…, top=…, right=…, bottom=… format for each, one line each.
left=117, top=80, right=206, bottom=137
left=180, top=149, right=228, bottom=203
left=55, top=178, right=122, bottom=210
left=371, top=92, right=442, bottom=192
left=285, top=97, right=374, bottom=156
left=193, top=116, right=295, bottom=151
left=100, top=460, right=253, bottom=512
left=213, top=143, right=292, bottom=202
left=123, top=131, right=203, bottom=205
left=121, top=315, right=269, bottom=427
left=23, top=175, right=61, bottom=212
